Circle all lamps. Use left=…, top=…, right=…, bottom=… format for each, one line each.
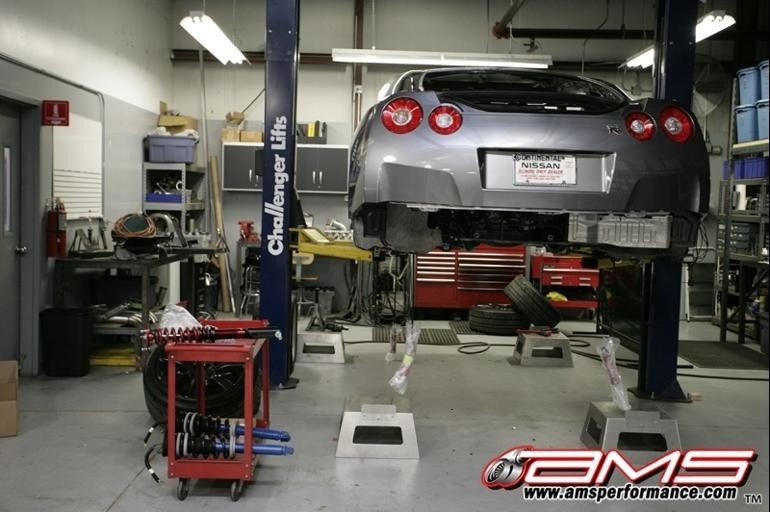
left=616, top=1, right=736, bottom=70
left=331, top=1, right=555, bottom=71
left=178, top=0, right=252, bottom=67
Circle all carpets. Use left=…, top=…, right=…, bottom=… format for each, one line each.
left=678, top=339, right=770, bottom=370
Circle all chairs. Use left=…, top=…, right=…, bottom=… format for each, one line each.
left=239, top=266, right=262, bottom=319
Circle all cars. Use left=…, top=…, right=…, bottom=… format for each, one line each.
left=343, top=65, right=712, bottom=264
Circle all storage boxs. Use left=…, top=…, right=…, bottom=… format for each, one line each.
left=143, top=135, right=199, bottom=164
left=757, top=312, right=769, bottom=354
left=159, top=101, right=197, bottom=136
left=0, top=360, right=19, bottom=438
left=723, top=60, right=769, bottom=180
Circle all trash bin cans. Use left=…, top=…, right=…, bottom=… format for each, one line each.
left=39, top=307, right=95, bottom=377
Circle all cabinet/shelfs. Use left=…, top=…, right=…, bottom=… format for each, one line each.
left=709, top=176, right=770, bottom=338
left=221, top=142, right=350, bottom=195
left=53, top=249, right=196, bottom=375
left=143, top=161, right=211, bottom=246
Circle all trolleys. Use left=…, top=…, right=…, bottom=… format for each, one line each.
left=164, top=310, right=272, bottom=503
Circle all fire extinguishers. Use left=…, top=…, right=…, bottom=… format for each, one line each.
left=46, top=197, right=67, bottom=258
